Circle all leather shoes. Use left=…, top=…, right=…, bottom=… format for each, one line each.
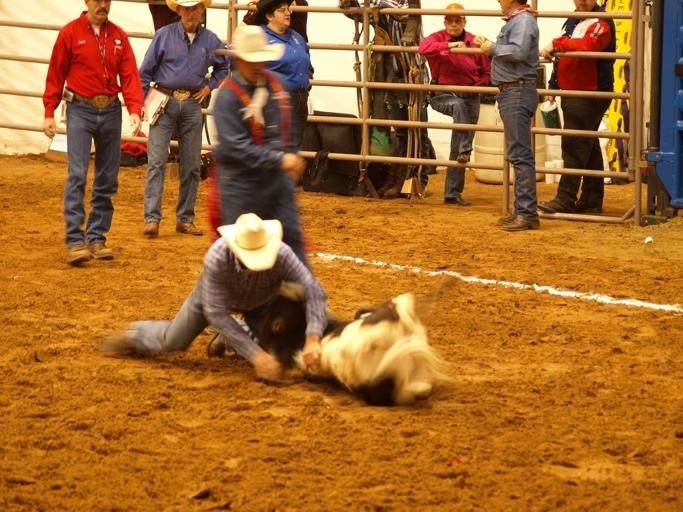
left=497, top=214, right=517, bottom=225
left=457, top=152, right=469, bottom=162
left=444, top=195, right=470, bottom=206
left=500, top=217, right=540, bottom=231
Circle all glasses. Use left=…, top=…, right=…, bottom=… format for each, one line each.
left=277, top=7, right=293, bottom=14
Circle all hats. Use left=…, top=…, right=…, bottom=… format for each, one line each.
left=443, top=4, right=464, bottom=19
left=215, top=212, right=284, bottom=272
left=165, top=0, right=212, bottom=12
left=215, top=25, right=286, bottom=63
left=254, top=0, right=294, bottom=27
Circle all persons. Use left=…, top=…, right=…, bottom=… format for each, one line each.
left=102, top=212, right=327, bottom=382
left=337, top=0, right=437, bottom=173
left=42, top=0, right=144, bottom=263
left=242, top=0, right=308, bottom=37
left=417, top=3, right=491, bottom=206
left=253, top=1, right=310, bottom=148
left=474, top=0, right=539, bottom=231
left=140, top=0, right=230, bottom=236
left=212, top=25, right=306, bottom=258
left=540, top=0, right=617, bottom=214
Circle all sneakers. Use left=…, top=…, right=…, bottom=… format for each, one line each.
left=206, top=332, right=224, bottom=359
left=575, top=199, right=603, bottom=214
left=175, top=222, right=203, bottom=235
left=538, top=196, right=575, bottom=214
left=67, top=245, right=90, bottom=263
left=143, top=220, right=159, bottom=236
left=88, top=242, right=114, bottom=260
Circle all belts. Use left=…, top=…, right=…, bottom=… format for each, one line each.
left=73, top=94, right=117, bottom=109
left=498, top=79, right=535, bottom=93
left=156, top=87, right=197, bottom=101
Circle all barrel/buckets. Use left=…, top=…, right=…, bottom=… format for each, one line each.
left=473, top=103, right=547, bottom=185
